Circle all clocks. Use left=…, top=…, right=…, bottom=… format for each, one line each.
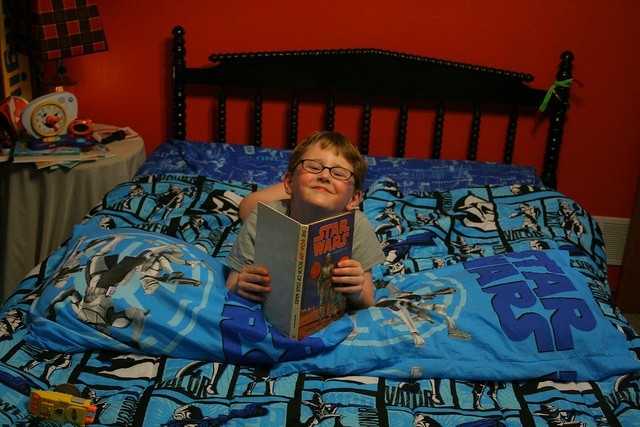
left=31, top=100, right=68, bottom=137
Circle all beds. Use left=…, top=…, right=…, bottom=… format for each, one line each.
left=0, top=24, right=640, bottom=427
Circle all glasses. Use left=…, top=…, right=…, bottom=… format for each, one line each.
left=291, top=158, right=357, bottom=181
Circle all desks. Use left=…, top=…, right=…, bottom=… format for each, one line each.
left=0, top=124, right=147, bottom=308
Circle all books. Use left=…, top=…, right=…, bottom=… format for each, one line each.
left=253, top=203, right=359, bottom=341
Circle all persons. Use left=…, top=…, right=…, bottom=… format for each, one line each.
left=226, top=129, right=375, bottom=310
